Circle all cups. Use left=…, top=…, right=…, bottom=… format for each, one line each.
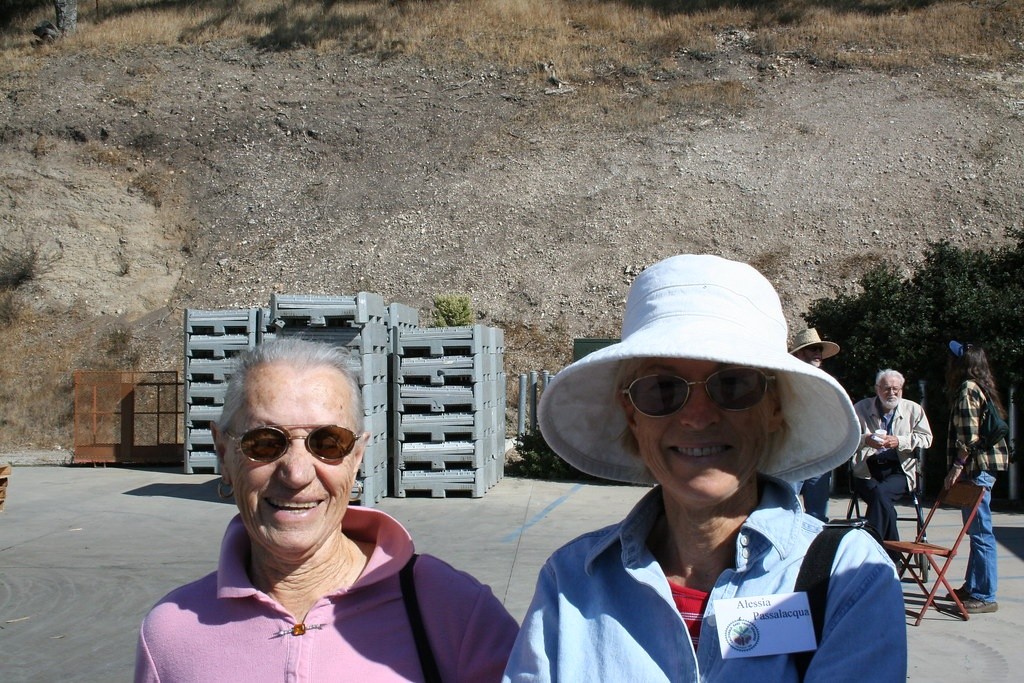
left=875, top=430, right=887, bottom=440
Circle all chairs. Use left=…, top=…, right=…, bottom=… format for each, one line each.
left=883, top=478, right=986, bottom=626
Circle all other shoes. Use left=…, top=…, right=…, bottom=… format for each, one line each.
left=945, top=587, right=971, bottom=601
left=954, top=597, right=997, bottom=613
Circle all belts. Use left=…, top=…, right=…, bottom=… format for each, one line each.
left=874, top=458, right=893, bottom=464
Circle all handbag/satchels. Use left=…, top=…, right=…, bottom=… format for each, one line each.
left=952, top=381, right=1008, bottom=451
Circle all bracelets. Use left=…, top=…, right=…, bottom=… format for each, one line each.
left=954, top=463, right=963, bottom=469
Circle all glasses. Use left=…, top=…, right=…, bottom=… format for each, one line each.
left=877, top=384, right=901, bottom=393
left=621, top=368, right=776, bottom=419
left=804, top=345, right=823, bottom=352
left=223, top=424, right=359, bottom=462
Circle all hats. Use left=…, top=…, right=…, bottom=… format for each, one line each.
left=788, top=327, right=841, bottom=360
left=537, top=254, right=860, bottom=486
left=949, top=340, right=974, bottom=360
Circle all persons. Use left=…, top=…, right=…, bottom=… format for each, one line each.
left=133, top=337, right=521, bottom=682
left=501, top=254, right=907, bottom=683
left=793, top=328, right=1008, bottom=613
